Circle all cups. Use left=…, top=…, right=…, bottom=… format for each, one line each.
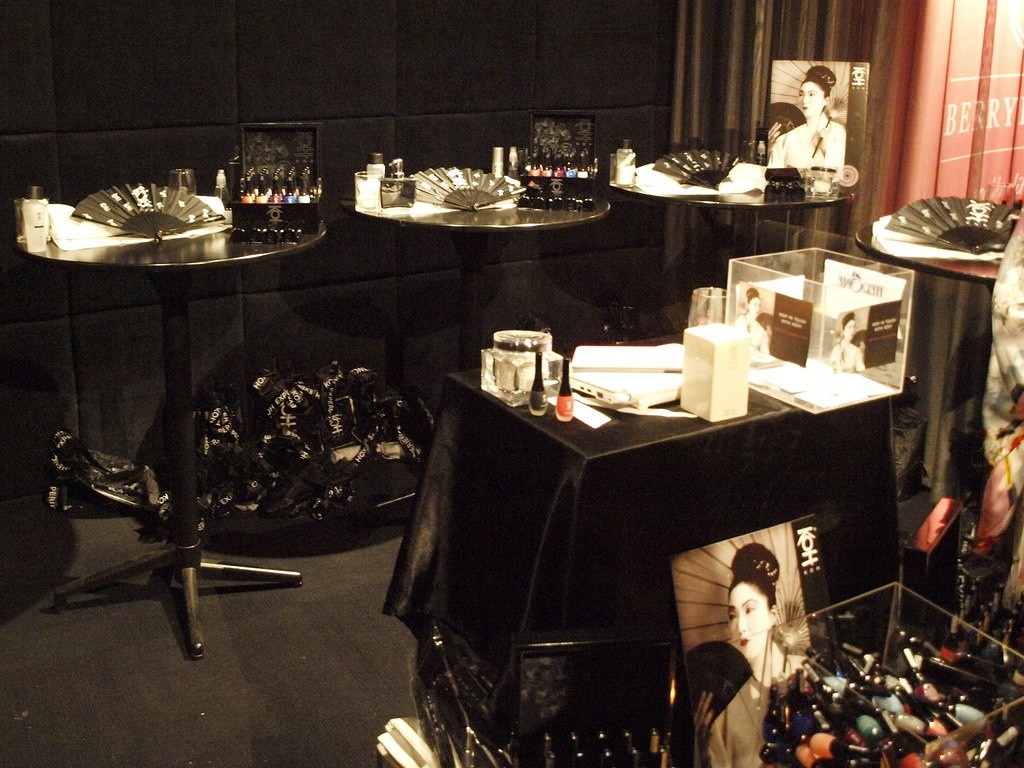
left=353, top=170, right=384, bottom=214
left=610, top=154, right=636, bottom=183
left=685, top=287, right=727, bottom=328
left=167, top=167, right=198, bottom=197
left=14, top=197, right=52, bottom=244
left=517, top=147, right=528, bottom=173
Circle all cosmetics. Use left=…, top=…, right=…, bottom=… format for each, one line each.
left=532, top=154, right=588, bottom=178
left=809, top=167, right=835, bottom=194
left=756, top=141, right=766, bottom=165
left=555, top=358, right=574, bottom=421
left=481, top=329, right=562, bottom=406
left=380, top=159, right=417, bottom=208
left=528, top=353, right=548, bottom=416
left=22, top=186, right=48, bottom=252
left=215, top=170, right=229, bottom=206
left=610, top=140, right=636, bottom=187
left=492, top=147, right=518, bottom=179
left=244, top=167, right=312, bottom=203
left=760, top=619, right=1024, bottom=768
left=367, top=153, right=385, bottom=177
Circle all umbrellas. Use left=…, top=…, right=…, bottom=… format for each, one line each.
left=771, top=62, right=850, bottom=159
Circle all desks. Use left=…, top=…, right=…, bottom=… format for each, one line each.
left=381, top=332, right=901, bottom=745
left=854, top=223, right=998, bottom=293
left=339, top=178, right=612, bottom=371
left=607, top=158, right=854, bottom=287
left=8, top=205, right=328, bottom=661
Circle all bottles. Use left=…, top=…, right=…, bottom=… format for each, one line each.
left=21, top=186, right=50, bottom=252
left=365, top=152, right=386, bottom=180
left=491, top=147, right=504, bottom=179
left=507, top=146, right=520, bottom=180
left=615, top=139, right=636, bottom=189
left=479, top=329, right=564, bottom=408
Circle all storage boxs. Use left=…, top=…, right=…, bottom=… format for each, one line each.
left=750, top=581, right=1024, bottom=768
left=517, top=108, right=599, bottom=213
left=723, top=245, right=915, bottom=415
left=231, top=122, right=322, bottom=235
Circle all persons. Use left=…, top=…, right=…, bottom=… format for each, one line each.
left=737, top=287, right=770, bottom=356
left=699, top=543, right=808, bottom=768
left=769, top=66, right=844, bottom=182
left=832, top=314, right=865, bottom=371
